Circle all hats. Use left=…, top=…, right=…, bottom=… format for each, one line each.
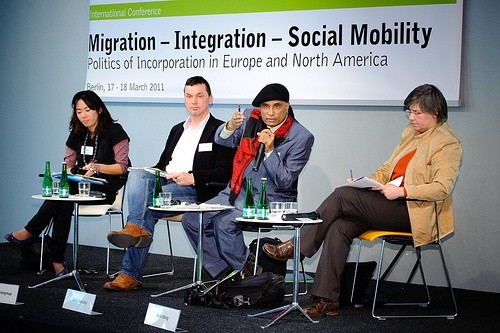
left=252, top=83, right=289, bottom=107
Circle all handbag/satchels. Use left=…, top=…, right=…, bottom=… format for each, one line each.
left=252, top=238, right=287, bottom=278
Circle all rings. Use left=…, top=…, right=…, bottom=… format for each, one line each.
left=175, top=177, right=177, bottom=180
left=233, top=117, right=236, bottom=119
left=268, top=134, right=270, bottom=137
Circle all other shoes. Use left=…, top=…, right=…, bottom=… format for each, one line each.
left=107, top=223, right=153, bottom=247
left=224, top=252, right=261, bottom=282
left=103, top=273, right=142, bottom=290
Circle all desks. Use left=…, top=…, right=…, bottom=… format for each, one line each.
left=28, top=195, right=106, bottom=292
left=235, top=213, right=323, bottom=328
left=147, top=202, right=235, bottom=297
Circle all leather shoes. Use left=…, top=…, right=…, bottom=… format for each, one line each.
left=263, top=239, right=305, bottom=262
left=301, top=297, right=339, bottom=318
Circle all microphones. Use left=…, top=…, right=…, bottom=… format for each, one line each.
left=71, top=168, right=98, bottom=177
left=282, top=211, right=321, bottom=221
left=252, top=142, right=264, bottom=173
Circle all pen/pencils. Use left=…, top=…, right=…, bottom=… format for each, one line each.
left=238, top=106, right=240, bottom=129
left=350, top=170, right=352, bottom=178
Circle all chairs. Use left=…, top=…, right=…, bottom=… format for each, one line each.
left=39, top=184, right=458, bottom=320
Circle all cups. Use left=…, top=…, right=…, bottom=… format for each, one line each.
left=159, top=191, right=172, bottom=207
left=270, top=201, right=297, bottom=215
left=78, top=181, right=90, bottom=196
left=53, top=179, right=59, bottom=193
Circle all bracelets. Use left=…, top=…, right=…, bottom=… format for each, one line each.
left=97, top=164, right=100, bottom=174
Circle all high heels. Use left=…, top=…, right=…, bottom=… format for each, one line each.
left=4, top=232, right=36, bottom=243
left=55, top=261, right=67, bottom=278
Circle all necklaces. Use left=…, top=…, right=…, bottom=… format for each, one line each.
left=83, top=134, right=98, bottom=165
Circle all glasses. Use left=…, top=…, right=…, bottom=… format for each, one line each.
left=406, top=109, right=428, bottom=117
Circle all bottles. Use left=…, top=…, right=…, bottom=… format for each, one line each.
left=243, top=177, right=255, bottom=218
left=152, top=170, right=164, bottom=208
left=42, top=161, right=53, bottom=196
left=59, top=162, right=69, bottom=197
left=257, top=178, right=269, bottom=219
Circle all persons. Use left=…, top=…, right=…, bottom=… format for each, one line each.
left=263, top=85, right=463, bottom=316
left=102, top=76, right=236, bottom=291
left=182, top=83, right=315, bottom=281
left=5, top=91, right=131, bottom=279
